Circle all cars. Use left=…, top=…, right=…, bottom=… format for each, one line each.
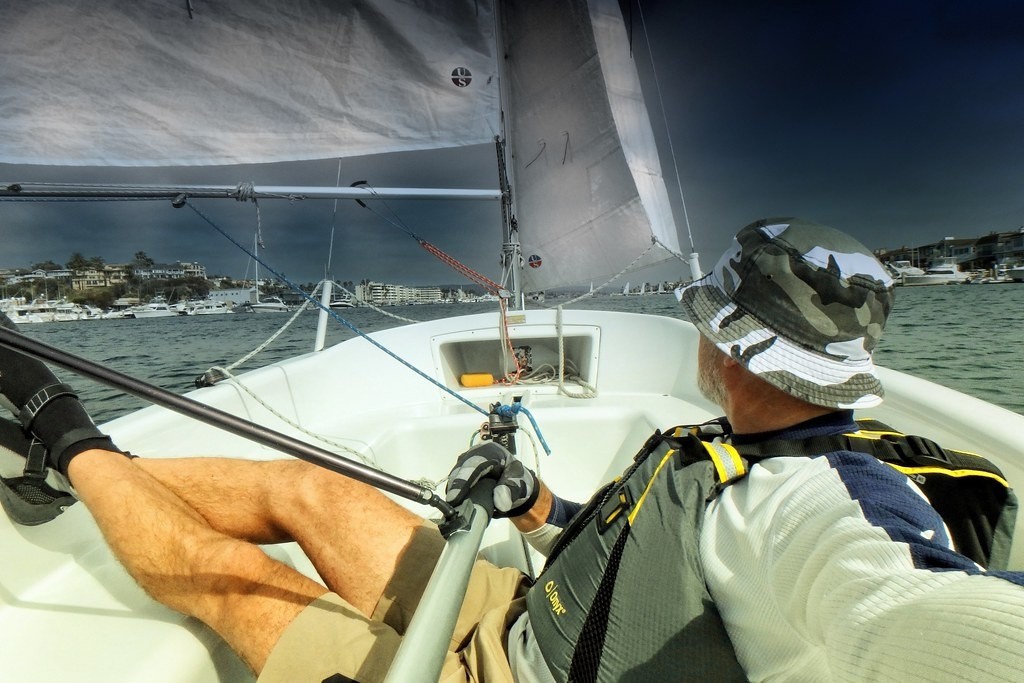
left=458, top=297, right=467, bottom=302
left=406, top=298, right=415, bottom=305
left=446, top=300, right=452, bottom=303
left=391, top=301, right=396, bottom=306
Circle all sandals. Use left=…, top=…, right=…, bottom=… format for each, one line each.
left=0, top=310, right=112, bottom=470
left=0, top=438, right=84, bottom=526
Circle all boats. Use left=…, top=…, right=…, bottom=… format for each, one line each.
left=169, top=300, right=229, bottom=315
left=131, top=303, right=177, bottom=317
left=981, top=269, right=1009, bottom=284
left=0, top=293, right=104, bottom=324
left=102, top=298, right=139, bottom=320
left=902, top=242, right=970, bottom=286
left=316, top=275, right=355, bottom=309
left=885, top=260, right=925, bottom=278
left=999, top=257, right=1024, bottom=282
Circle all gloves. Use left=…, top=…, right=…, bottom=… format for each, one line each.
left=445, top=440, right=540, bottom=518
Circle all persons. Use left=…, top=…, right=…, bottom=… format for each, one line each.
left=0, top=216, right=1024, bottom=683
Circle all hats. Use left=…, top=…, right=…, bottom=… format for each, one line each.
left=675, top=216, right=894, bottom=409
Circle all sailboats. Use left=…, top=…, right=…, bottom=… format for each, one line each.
left=589, top=281, right=597, bottom=299
left=623, top=282, right=631, bottom=296
left=243, top=232, right=292, bottom=313
left=656, top=283, right=666, bottom=293
